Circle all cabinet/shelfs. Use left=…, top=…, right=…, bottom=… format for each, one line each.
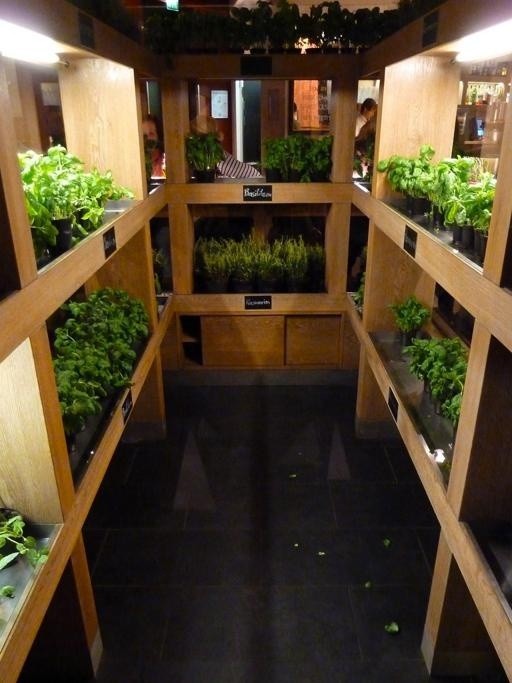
left=0, top=1, right=174, bottom=682
left=345, top=7, right=509, bottom=679
left=178, top=47, right=354, bottom=367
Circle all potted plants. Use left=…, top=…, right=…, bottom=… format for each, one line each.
left=14, top=144, right=135, bottom=254
left=378, top=143, right=494, bottom=260
left=389, top=299, right=467, bottom=435
left=190, top=234, right=323, bottom=294
left=184, top=129, right=221, bottom=181
left=0, top=499, right=51, bottom=567
left=51, top=288, right=150, bottom=475
left=254, top=132, right=333, bottom=181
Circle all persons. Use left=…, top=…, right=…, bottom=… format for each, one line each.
left=143, top=115, right=166, bottom=177
left=354, top=98, right=378, bottom=140
left=190, top=115, right=261, bottom=178
